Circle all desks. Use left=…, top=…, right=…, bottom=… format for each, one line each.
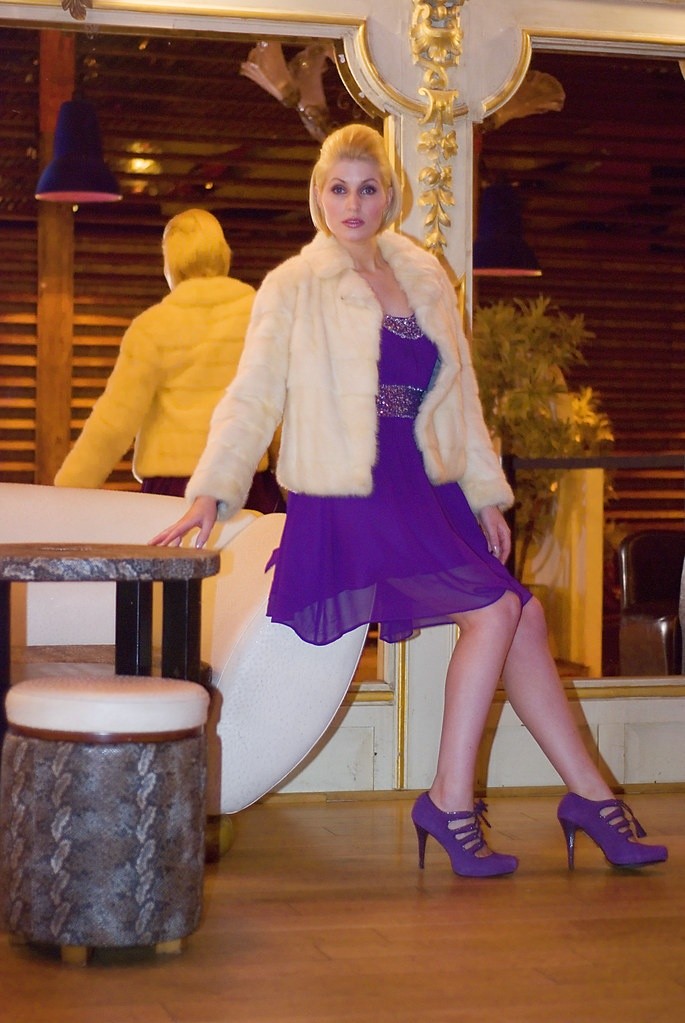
left=0, top=542, right=221, bottom=744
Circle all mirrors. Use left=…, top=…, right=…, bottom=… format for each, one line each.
left=470, top=27, right=685, bottom=703
left=0, top=0, right=399, bottom=706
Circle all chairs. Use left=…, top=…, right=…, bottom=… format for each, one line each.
left=616, top=529, right=685, bottom=675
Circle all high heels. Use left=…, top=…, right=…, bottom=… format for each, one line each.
left=412, top=788, right=519, bottom=879
left=556, top=792, right=669, bottom=872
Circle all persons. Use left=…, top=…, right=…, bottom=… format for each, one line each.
left=54, top=209, right=290, bottom=514
left=148, top=125, right=668, bottom=876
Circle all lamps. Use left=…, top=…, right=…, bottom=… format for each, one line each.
left=34, top=33, right=124, bottom=201
left=237, top=39, right=566, bottom=145
left=472, top=182, right=542, bottom=277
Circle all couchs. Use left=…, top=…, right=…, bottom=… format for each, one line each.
left=0, top=481, right=371, bottom=864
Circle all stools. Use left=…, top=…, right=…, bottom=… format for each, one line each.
left=0, top=676, right=210, bottom=967
